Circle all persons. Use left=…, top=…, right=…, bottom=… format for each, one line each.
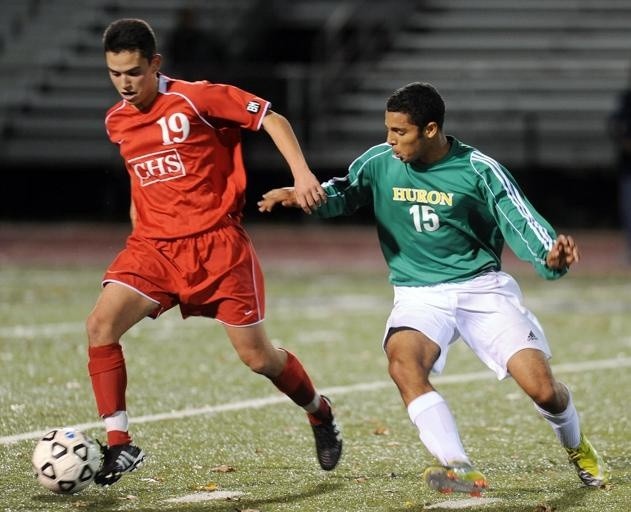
left=254, top=81, right=612, bottom=498
left=85, top=17, right=344, bottom=489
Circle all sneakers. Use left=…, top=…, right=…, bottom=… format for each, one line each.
left=94, top=442, right=145, bottom=486
left=561, top=434, right=612, bottom=486
left=425, top=464, right=486, bottom=496
left=306, top=395, right=342, bottom=471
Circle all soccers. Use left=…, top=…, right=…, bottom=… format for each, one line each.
left=32, top=429, right=100, bottom=493
